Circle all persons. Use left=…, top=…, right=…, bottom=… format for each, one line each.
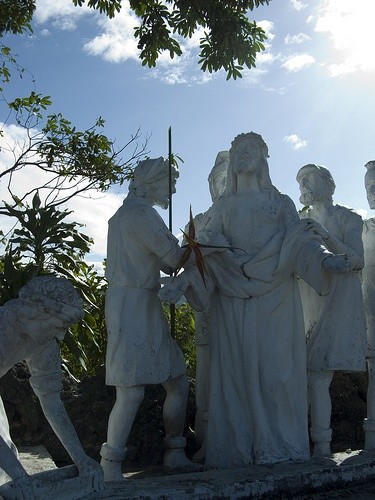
left=0, top=275, right=105, bottom=500
left=100, top=131, right=375, bottom=482
